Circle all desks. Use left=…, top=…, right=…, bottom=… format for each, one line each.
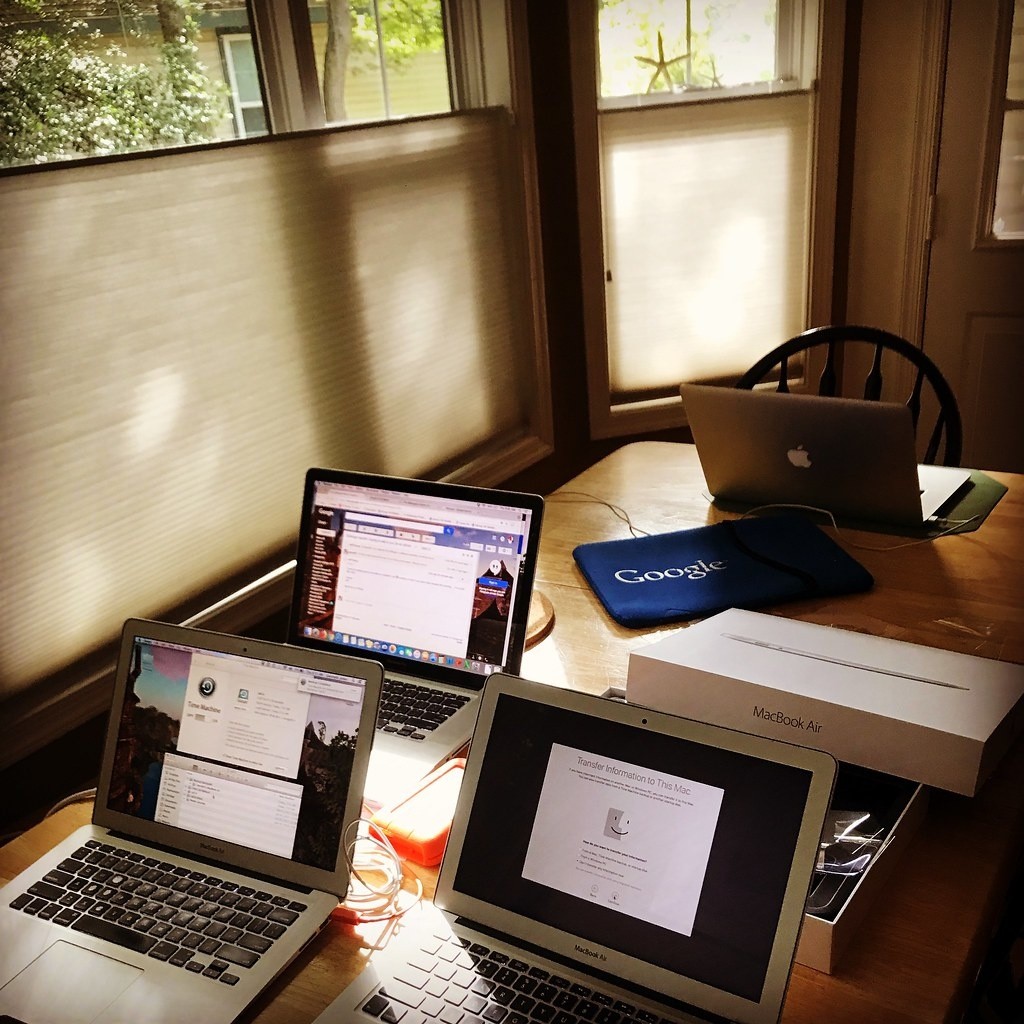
left=0, top=441, right=1024, bottom=1024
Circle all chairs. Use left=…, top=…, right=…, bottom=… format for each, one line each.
left=732, top=323, right=964, bottom=469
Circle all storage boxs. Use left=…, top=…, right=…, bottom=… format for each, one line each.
left=598, top=607, right=1024, bottom=975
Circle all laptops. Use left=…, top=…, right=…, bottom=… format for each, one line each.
left=679, top=383, right=972, bottom=531
left=0, top=466, right=837, bottom=1024
